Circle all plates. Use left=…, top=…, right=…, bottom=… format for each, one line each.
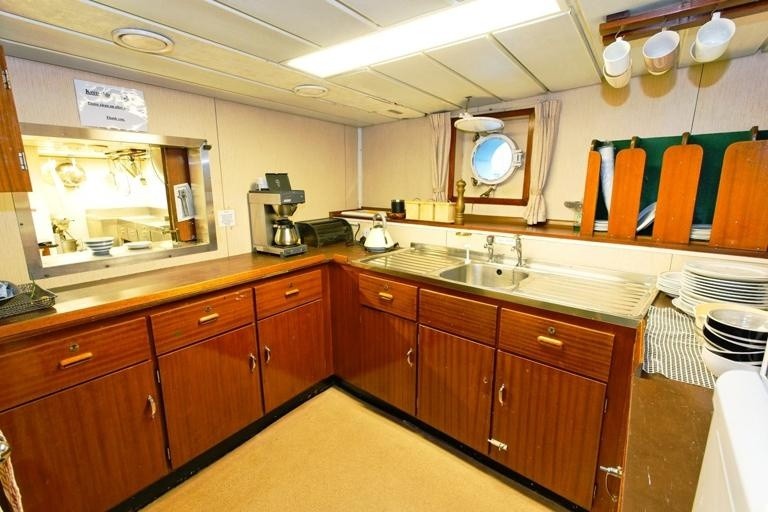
left=635, top=201, right=657, bottom=234
left=122, top=239, right=151, bottom=249
left=593, top=218, right=609, bottom=233
left=690, top=223, right=710, bottom=241
left=653, top=258, right=768, bottom=316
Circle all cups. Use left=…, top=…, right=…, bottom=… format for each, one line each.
left=686, top=9, right=737, bottom=63
left=390, top=199, right=405, bottom=213
left=598, top=36, right=633, bottom=92
left=641, top=28, right=681, bottom=78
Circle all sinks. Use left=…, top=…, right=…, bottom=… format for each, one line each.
left=440, top=262, right=529, bottom=289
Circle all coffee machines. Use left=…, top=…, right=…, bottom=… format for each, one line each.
left=246, top=171, right=309, bottom=258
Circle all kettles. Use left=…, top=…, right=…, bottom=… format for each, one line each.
left=361, top=212, right=394, bottom=253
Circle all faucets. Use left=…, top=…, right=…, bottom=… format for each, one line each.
left=484, top=235, right=494, bottom=261
left=510, top=234, right=522, bottom=267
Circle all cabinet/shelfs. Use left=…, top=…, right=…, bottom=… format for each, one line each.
left=357, top=272, right=418, bottom=421
left=0, top=315, right=170, bottom=512
left=150, top=265, right=334, bottom=470
left=414, top=288, right=615, bottom=511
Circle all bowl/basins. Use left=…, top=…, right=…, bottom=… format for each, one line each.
left=82, top=236, right=113, bottom=256
left=0, top=281, right=23, bottom=307
left=690, top=301, right=767, bottom=378
